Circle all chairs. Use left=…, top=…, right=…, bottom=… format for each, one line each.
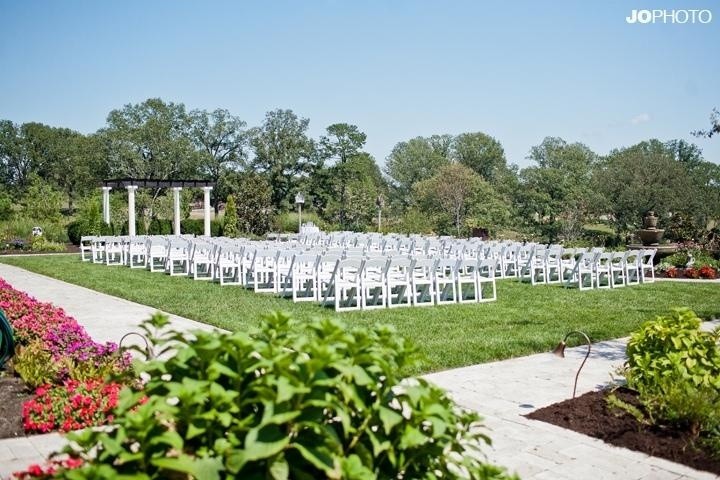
left=78, top=223, right=658, bottom=313
